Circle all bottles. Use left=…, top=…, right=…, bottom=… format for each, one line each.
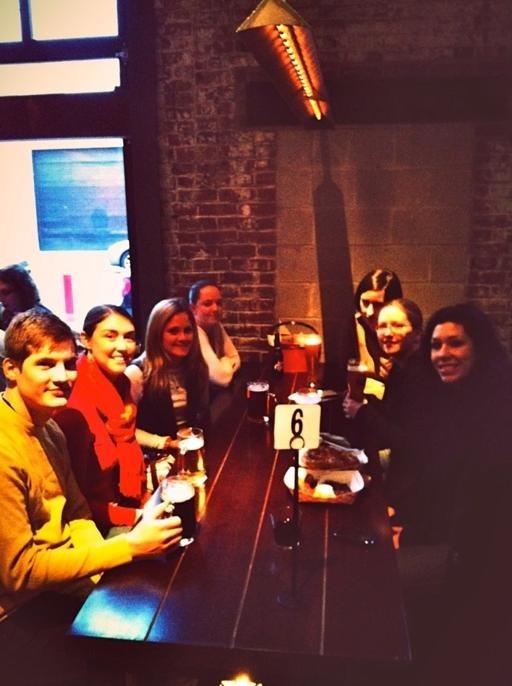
left=272, top=329, right=283, bottom=384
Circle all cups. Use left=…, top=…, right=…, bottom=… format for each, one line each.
left=345, top=358, right=368, bottom=406
left=303, top=334, right=323, bottom=389
left=159, top=425, right=208, bottom=548
left=246, top=380, right=277, bottom=421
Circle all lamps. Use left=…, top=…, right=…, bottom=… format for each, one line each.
left=237, top=0, right=331, bottom=123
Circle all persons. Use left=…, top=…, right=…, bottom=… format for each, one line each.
left=2, top=266, right=241, bottom=685
left=341, top=267, right=512, bottom=685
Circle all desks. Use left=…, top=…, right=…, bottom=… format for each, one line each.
left=66, top=363, right=416, bottom=671
